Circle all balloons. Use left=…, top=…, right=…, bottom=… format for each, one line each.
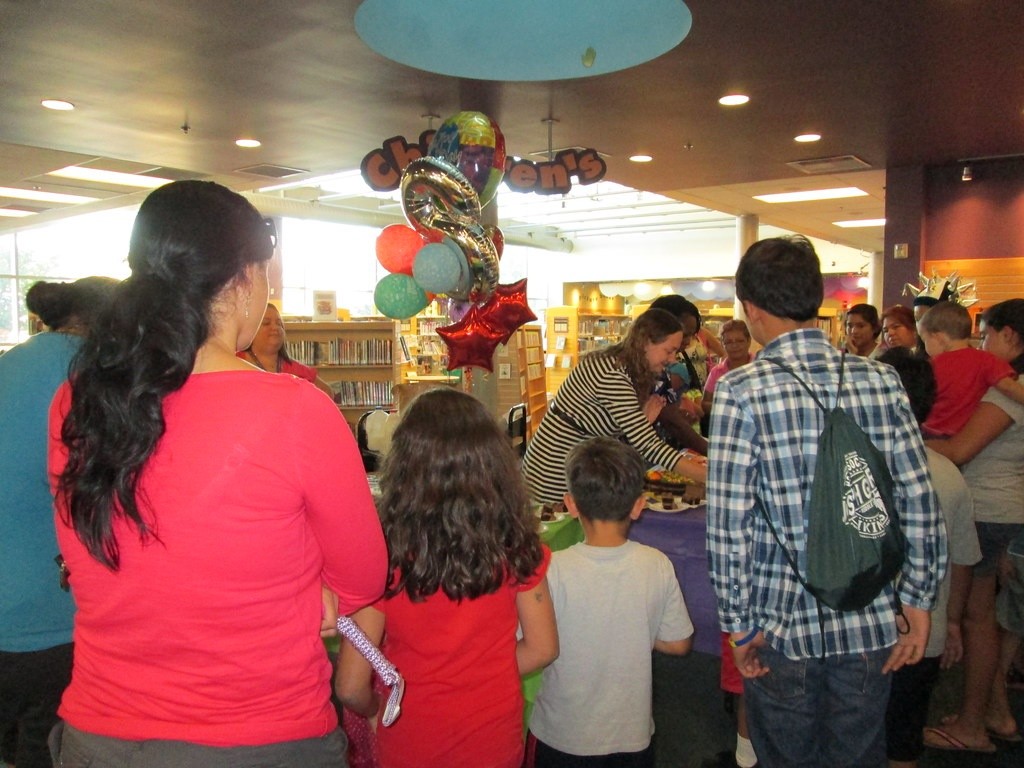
left=373, top=110, right=537, bottom=373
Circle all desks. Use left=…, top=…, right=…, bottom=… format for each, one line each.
left=629, top=502, right=724, bottom=656
left=325, top=507, right=583, bottom=750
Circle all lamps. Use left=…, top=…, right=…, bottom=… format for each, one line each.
left=961, top=165, right=973, bottom=181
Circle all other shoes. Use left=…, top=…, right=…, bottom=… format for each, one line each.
left=649, top=718, right=655, bottom=736
left=736, top=733, right=758, bottom=768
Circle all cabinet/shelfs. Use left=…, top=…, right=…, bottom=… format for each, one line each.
left=284, top=302, right=846, bottom=446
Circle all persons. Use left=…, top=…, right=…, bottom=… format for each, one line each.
left=667, top=361, right=690, bottom=408
left=914, top=282, right=959, bottom=359
left=844, top=304, right=889, bottom=360
left=918, top=301, right=1024, bottom=441
left=701, top=319, right=754, bottom=415
left=874, top=347, right=983, bottom=768
left=236, top=303, right=334, bottom=402
left=881, top=306, right=919, bottom=356
left=0, top=276, right=124, bottom=768
left=706, top=235, right=948, bottom=768
left=923, top=298, right=1024, bottom=768
left=650, top=295, right=708, bottom=457
left=46, top=178, right=389, bottom=768
left=516, top=437, right=695, bottom=768
left=519, top=308, right=706, bottom=506
left=336, top=388, right=559, bottom=768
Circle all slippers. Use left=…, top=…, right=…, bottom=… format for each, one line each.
left=923, top=728, right=997, bottom=752
left=936, top=714, right=1021, bottom=741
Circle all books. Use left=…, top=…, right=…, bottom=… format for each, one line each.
left=516, top=331, right=543, bottom=394
left=578, top=320, right=629, bottom=360
left=284, top=338, right=393, bottom=406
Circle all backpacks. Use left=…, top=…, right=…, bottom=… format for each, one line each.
left=753, top=350, right=909, bottom=611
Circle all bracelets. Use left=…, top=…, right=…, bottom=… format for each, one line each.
left=729, top=629, right=757, bottom=648
